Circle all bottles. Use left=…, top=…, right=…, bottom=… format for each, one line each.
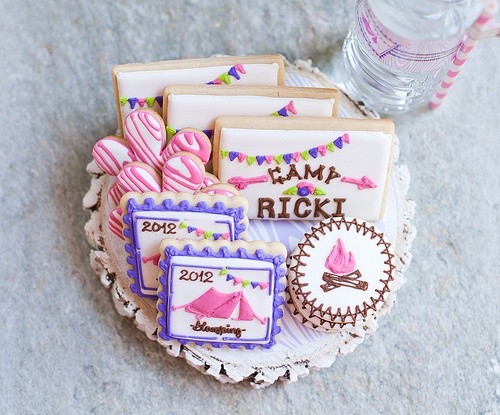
left=341, top=0, right=484, bottom=115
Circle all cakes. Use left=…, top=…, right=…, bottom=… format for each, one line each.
left=80, top=54, right=417, bottom=389
